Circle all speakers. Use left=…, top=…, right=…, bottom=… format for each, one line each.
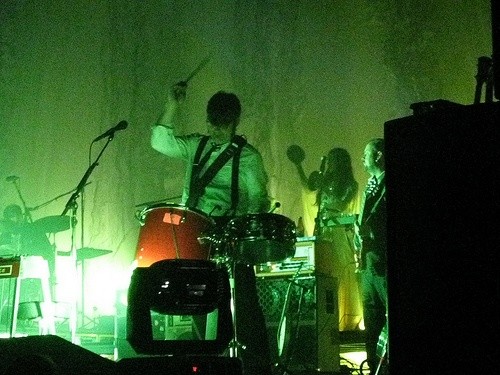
left=383, top=101, right=500, bottom=375
left=254, top=274, right=339, bottom=375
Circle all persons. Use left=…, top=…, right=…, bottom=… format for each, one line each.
left=151, top=81, right=275, bottom=375
left=297, top=147, right=364, bottom=332
left=3, top=204, right=23, bottom=223
left=352, top=137, right=388, bottom=370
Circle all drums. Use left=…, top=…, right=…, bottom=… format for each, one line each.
left=226, top=213, right=296, bottom=263
left=129, top=201, right=217, bottom=270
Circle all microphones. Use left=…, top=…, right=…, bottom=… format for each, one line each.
left=94, top=120, right=128, bottom=140
left=6, top=175, right=16, bottom=182
